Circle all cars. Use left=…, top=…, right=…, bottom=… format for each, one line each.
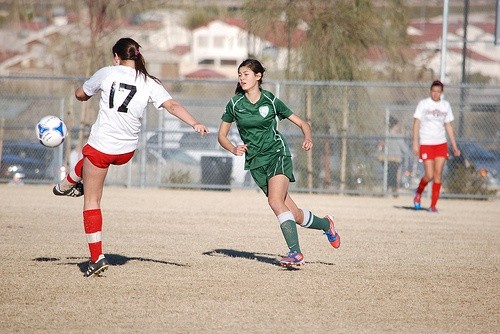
left=0, top=130, right=500, bottom=195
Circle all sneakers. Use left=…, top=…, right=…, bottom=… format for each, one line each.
left=323, top=216, right=340, bottom=250
left=83, top=254, right=109, bottom=278
left=280, top=250, right=305, bottom=270
left=53, top=183, right=84, bottom=197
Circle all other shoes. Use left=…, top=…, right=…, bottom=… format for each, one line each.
left=414, top=196, right=420, bottom=209
left=428, top=207, right=438, bottom=213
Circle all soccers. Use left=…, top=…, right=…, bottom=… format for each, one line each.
left=35, top=116, right=68, bottom=147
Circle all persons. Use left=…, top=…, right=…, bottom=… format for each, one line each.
left=377, top=115, right=412, bottom=198
left=53, top=37, right=209, bottom=277
left=217, top=59, right=340, bottom=266
left=412, top=80, right=461, bottom=213
